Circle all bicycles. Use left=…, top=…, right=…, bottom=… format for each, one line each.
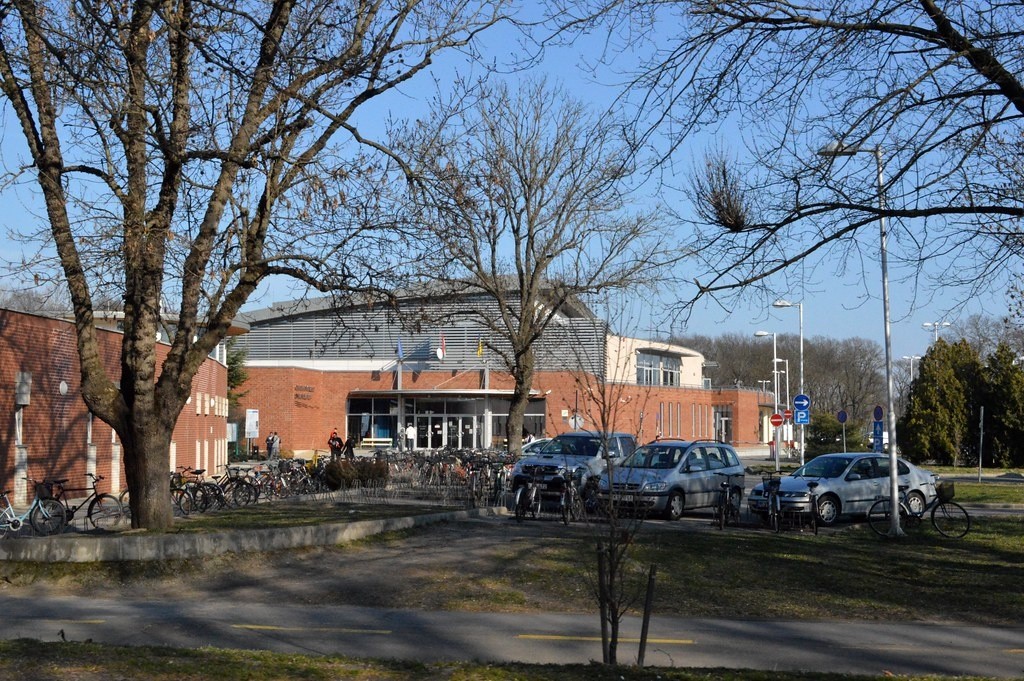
left=759, top=470, right=784, bottom=533
left=515, top=464, right=551, bottom=523
left=32, top=473, right=122, bottom=536
left=0, top=477, right=66, bottom=540
left=794, top=473, right=829, bottom=536
left=119, top=445, right=519, bottom=519
left=713, top=472, right=744, bottom=530
left=868, top=473, right=970, bottom=539
left=555, top=467, right=587, bottom=526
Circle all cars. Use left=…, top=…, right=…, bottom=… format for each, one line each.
left=748, top=452, right=937, bottom=527
left=520, top=438, right=576, bottom=459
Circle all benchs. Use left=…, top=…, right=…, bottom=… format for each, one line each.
left=688, top=452, right=722, bottom=470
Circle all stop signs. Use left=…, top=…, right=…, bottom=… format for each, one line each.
left=784, top=410, right=791, bottom=419
left=771, top=415, right=783, bottom=426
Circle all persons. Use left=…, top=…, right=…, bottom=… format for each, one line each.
left=405, top=423, right=415, bottom=451
left=329, top=428, right=355, bottom=463
left=265, top=431, right=281, bottom=462
left=502, top=424, right=535, bottom=450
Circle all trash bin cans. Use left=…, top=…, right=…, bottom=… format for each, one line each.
left=252, top=446, right=259, bottom=461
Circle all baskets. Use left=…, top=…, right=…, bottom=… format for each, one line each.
left=935, top=480, right=955, bottom=502
left=34, top=482, right=53, bottom=500
left=762, top=475, right=781, bottom=491
left=529, top=468, right=545, bottom=482
left=228, top=467, right=239, bottom=481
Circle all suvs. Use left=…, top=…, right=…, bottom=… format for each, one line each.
left=512, top=432, right=642, bottom=514
left=597, top=438, right=745, bottom=521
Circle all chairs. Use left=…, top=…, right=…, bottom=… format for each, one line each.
left=654, top=453, right=668, bottom=468
left=831, top=462, right=871, bottom=479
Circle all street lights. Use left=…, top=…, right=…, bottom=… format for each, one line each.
left=902, top=356, right=922, bottom=405
left=772, top=299, right=805, bottom=476
left=772, top=370, right=785, bottom=441
left=817, top=141, right=899, bottom=543
left=923, top=322, right=951, bottom=392
left=754, top=330, right=779, bottom=472
left=758, top=380, right=771, bottom=404
left=772, top=358, right=791, bottom=459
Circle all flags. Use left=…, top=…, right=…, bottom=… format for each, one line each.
left=477, top=334, right=482, bottom=357
left=442, top=330, right=446, bottom=357
left=398, top=335, right=404, bottom=361
